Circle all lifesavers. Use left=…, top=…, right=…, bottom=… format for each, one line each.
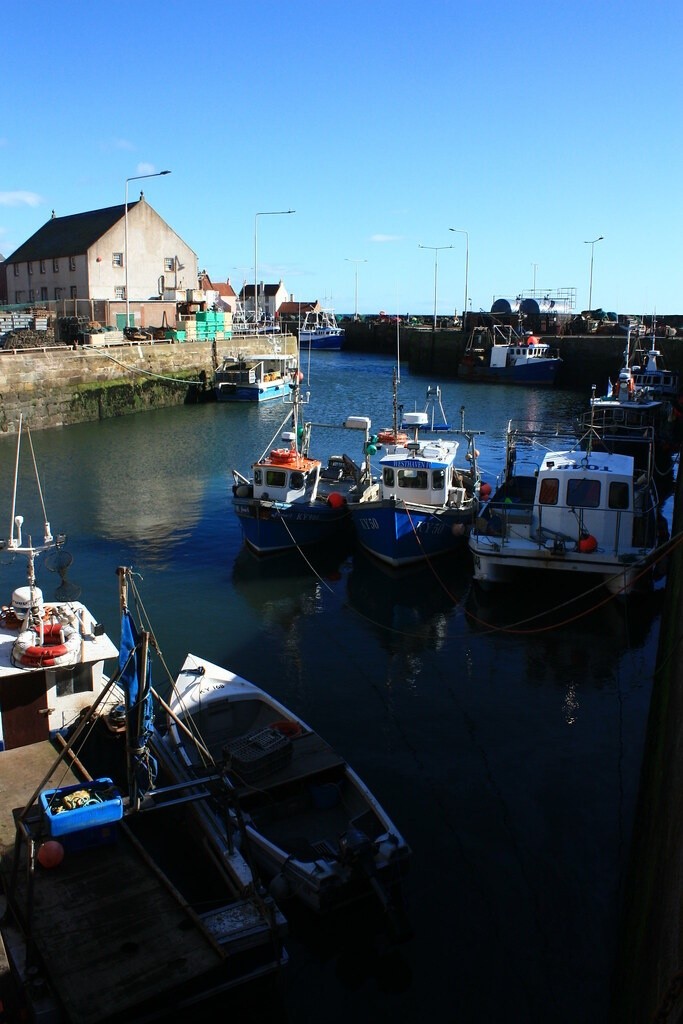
left=379, top=432, right=407, bottom=443
left=269, top=449, right=297, bottom=462
left=12, top=624, right=82, bottom=666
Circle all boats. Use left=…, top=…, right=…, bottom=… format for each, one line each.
left=214, top=336, right=303, bottom=404
left=165, top=647, right=425, bottom=931
left=292, top=307, right=345, bottom=352
left=230, top=303, right=362, bottom=561
left=574, top=326, right=681, bottom=474
left=466, top=391, right=663, bottom=622
left=347, top=316, right=490, bottom=578
left=2, top=412, right=296, bottom=1024
left=465, top=319, right=563, bottom=387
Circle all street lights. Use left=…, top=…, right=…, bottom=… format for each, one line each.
left=345, top=259, right=369, bottom=320
left=253, top=209, right=297, bottom=334
left=531, top=263, right=538, bottom=298
left=449, top=228, right=469, bottom=319
left=232, top=267, right=253, bottom=320
left=417, top=244, right=456, bottom=330
left=124, top=169, right=172, bottom=329
left=585, top=236, right=605, bottom=312
